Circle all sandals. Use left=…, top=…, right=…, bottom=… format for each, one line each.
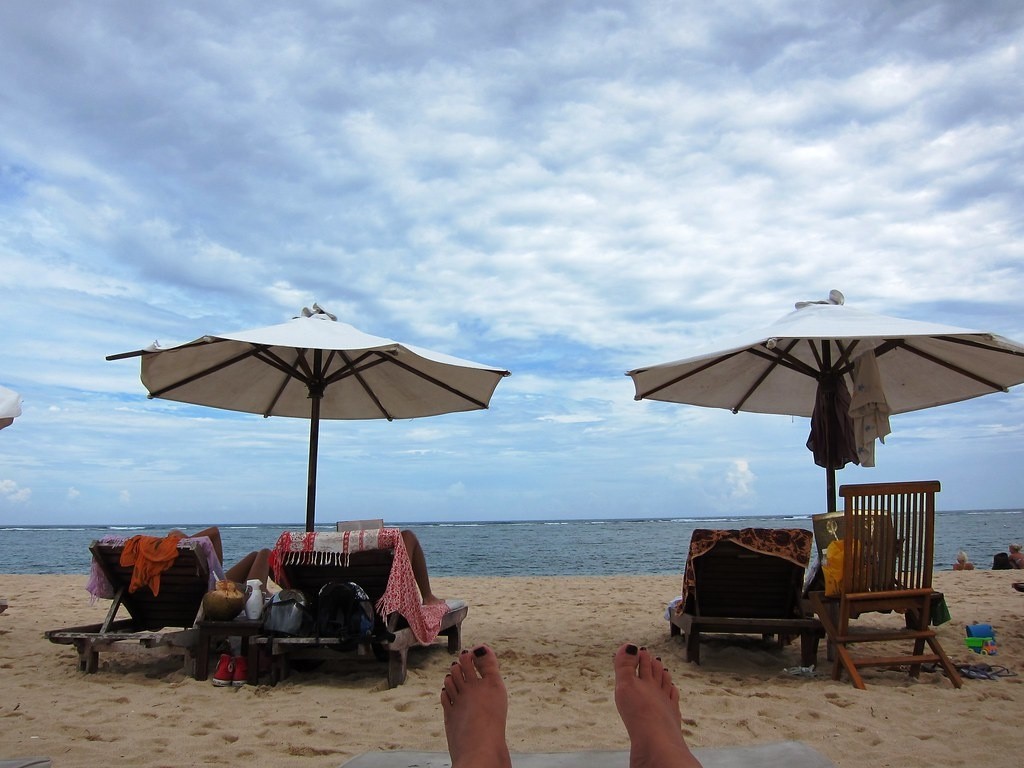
left=785, top=664, right=817, bottom=678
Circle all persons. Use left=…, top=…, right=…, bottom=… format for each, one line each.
left=1008, top=543, right=1024, bottom=569
left=953, top=551, right=975, bottom=570
left=440, top=642, right=703, bottom=768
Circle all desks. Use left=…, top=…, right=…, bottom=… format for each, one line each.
left=195, top=618, right=272, bottom=685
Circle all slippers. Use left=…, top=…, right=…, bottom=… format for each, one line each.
left=960, top=662, right=1018, bottom=679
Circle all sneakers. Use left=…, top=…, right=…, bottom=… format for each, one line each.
left=212, top=653, right=248, bottom=686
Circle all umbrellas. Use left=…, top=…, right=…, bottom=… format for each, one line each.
left=625, top=289, right=1024, bottom=514
left=106, top=302, right=512, bottom=532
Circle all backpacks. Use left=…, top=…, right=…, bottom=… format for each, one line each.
left=262, top=588, right=310, bottom=634
left=315, top=580, right=375, bottom=651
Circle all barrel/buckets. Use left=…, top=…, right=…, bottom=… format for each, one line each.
left=966, top=623, right=996, bottom=655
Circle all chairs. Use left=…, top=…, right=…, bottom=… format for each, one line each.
left=44, top=541, right=226, bottom=677
left=670, top=526, right=826, bottom=670
left=250, top=528, right=469, bottom=689
left=800, top=479, right=963, bottom=690
left=336, top=518, right=384, bottom=531
